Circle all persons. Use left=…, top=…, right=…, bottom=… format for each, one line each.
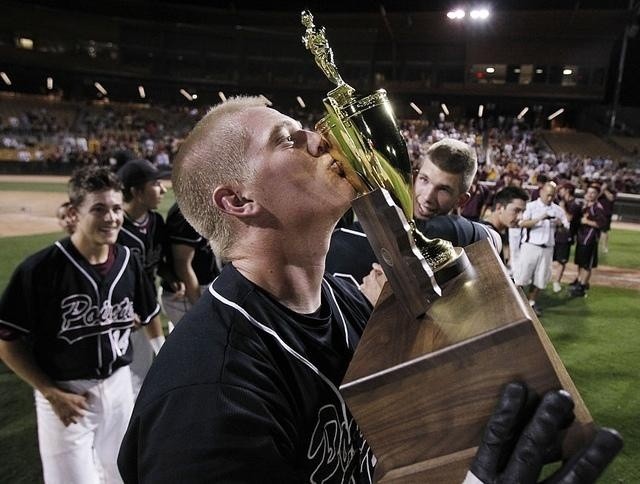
left=118, top=93, right=624, bottom=484
left=1, top=160, right=218, bottom=483
left=2, top=95, right=130, bottom=165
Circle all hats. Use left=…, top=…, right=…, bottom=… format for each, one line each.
left=118, top=160, right=171, bottom=190
left=558, top=183, right=576, bottom=191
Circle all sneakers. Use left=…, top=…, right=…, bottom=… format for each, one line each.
left=553, top=282, right=561, bottom=292
left=569, top=277, right=590, bottom=298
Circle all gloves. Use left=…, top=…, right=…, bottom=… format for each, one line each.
left=461, top=379, right=624, bottom=483
